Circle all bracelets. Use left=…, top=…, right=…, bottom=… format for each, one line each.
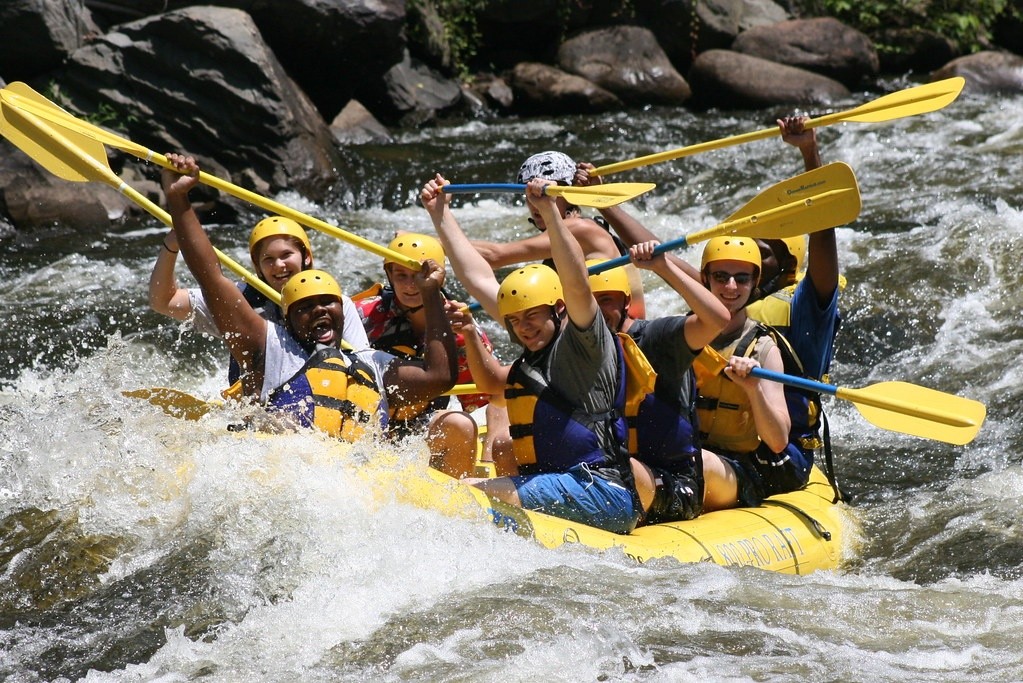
left=162, top=240, right=178, bottom=253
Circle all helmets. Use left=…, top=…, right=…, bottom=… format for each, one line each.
left=700, top=235, right=762, bottom=288
left=515, top=151, right=578, bottom=187
left=383, top=233, right=446, bottom=285
left=248, top=215, right=315, bottom=279
left=280, top=269, right=343, bottom=323
left=781, top=235, right=806, bottom=281
left=496, top=263, right=566, bottom=317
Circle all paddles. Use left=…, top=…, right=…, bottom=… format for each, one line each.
left=440, top=180, right=658, bottom=211
left=755, top=366, right=988, bottom=447
left=0, top=87, right=422, bottom=271
left=1, top=80, right=352, bottom=353
left=123, top=383, right=477, bottom=423
left=588, top=75, right=967, bottom=178
left=458, top=161, right=863, bottom=314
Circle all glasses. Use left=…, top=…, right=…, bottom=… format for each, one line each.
left=711, top=270, right=753, bottom=285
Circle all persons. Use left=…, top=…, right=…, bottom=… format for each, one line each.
left=149, top=113, right=840, bottom=535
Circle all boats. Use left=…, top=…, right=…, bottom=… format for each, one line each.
left=160, top=431, right=864, bottom=578
